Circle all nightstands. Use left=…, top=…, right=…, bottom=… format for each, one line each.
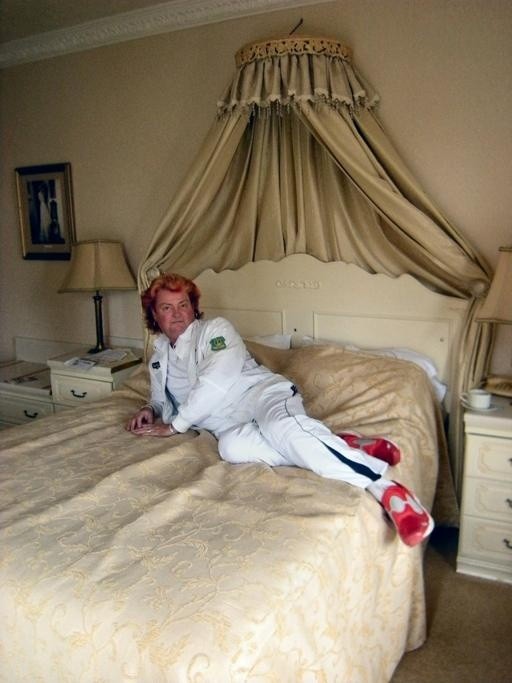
left=455, top=394, right=511, bottom=586
left=0, top=336, right=145, bottom=432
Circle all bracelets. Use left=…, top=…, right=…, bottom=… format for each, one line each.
left=168, top=423, right=176, bottom=435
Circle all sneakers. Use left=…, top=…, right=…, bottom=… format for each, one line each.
left=335, top=430, right=401, bottom=465
left=379, top=480, right=435, bottom=548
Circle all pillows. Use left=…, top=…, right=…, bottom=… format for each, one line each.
left=241, top=333, right=293, bottom=351
left=301, top=335, right=438, bottom=379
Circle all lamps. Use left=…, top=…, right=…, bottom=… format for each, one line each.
left=57, top=238, right=138, bottom=355
left=474, top=246, right=512, bottom=325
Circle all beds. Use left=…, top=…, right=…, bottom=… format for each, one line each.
left=0, top=253, right=494, bottom=683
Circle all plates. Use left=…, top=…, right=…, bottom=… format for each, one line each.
left=460, top=401, right=501, bottom=414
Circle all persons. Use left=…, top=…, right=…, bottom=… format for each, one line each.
left=123, top=269, right=436, bottom=548
left=31, top=183, right=63, bottom=244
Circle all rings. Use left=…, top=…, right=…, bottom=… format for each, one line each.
left=147, top=429, right=152, bottom=432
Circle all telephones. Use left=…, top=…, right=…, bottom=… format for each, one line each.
left=484, top=375, right=512, bottom=398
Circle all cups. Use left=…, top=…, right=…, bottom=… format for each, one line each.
left=459, top=389, right=492, bottom=409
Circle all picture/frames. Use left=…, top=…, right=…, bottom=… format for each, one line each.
left=15, top=162, right=76, bottom=260
